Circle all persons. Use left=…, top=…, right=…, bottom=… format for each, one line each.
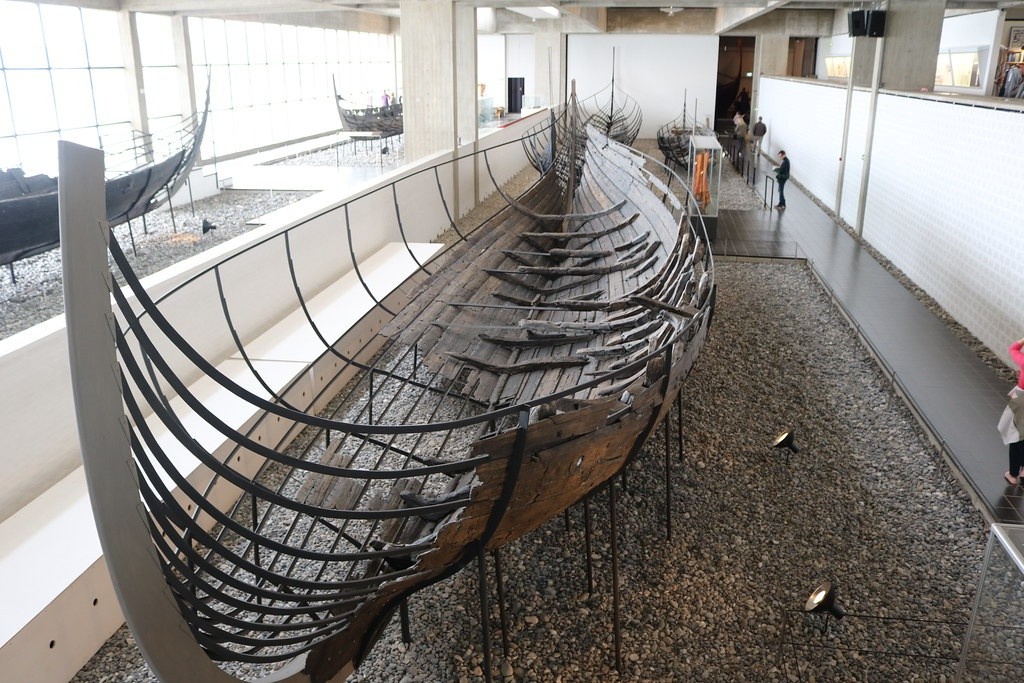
left=732, top=111, right=745, bottom=140
left=996, top=337, right=1024, bottom=485
left=737, top=88, right=748, bottom=112
left=391, top=92, right=396, bottom=105
left=735, top=117, right=747, bottom=156
left=381, top=90, right=390, bottom=107
left=773, top=150, right=790, bottom=209
left=751, top=116, right=766, bottom=157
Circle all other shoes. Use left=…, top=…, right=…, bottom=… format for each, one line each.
left=1005, top=471, right=1018, bottom=484
left=1019, top=466, right=1024, bottom=477
left=774, top=205, right=785, bottom=209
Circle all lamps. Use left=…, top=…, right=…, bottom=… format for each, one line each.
left=804, top=580, right=846, bottom=620
left=773, top=428, right=799, bottom=466
left=203, top=219, right=217, bottom=239
left=660, top=6, right=684, bottom=17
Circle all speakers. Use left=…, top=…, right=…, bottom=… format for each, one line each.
left=866, top=10, right=886, bottom=37
left=848, top=10, right=866, bottom=36
